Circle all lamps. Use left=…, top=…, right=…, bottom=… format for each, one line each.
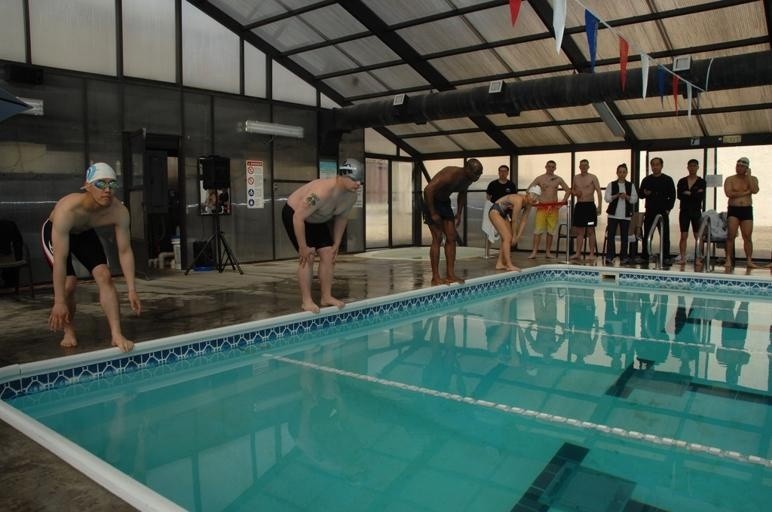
left=392, top=93, right=406, bottom=107
left=673, top=55, right=693, bottom=74
left=245, top=120, right=305, bottom=140
left=488, top=80, right=504, bottom=94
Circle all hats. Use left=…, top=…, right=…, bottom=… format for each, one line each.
left=528, top=184, right=542, bottom=196
left=338, top=159, right=364, bottom=180
left=86, top=162, right=117, bottom=183
left=737, top=157, right=749, bottom=167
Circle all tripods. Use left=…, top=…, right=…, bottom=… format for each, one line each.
left=183, top=190, right=244, bottom=275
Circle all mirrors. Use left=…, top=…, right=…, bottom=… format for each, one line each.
left=199, top=159, right=231, bottom=216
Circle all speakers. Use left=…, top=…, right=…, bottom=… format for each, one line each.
left=202, top=155, right=231, bottom=191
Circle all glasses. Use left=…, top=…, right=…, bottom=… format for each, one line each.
left=94, top=180, right=118, bottom=190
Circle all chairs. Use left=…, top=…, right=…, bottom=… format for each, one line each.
left=601, top=213, right=646, bottom=260
left=555, top=213, right=598, bottom=260
left=1, top=221, right=38, bottom=302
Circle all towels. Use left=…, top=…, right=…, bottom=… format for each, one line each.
left=481, top=201, right=505, bottom=245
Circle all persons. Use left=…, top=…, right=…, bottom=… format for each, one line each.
left=282, top=156, right=364, bottom=313
left=488, top=186, right=543, bottom=273
left=289, top=341, right=349, bottom=460
left=217, top=188, right=230, bottom=213
left=204, top=189, right=220, bottom=215
left=484, top=164, right=516, bottom=260
left=721, top=157, right=758, bottom=268
left=636, top=157, right=675, bottom=267
left=526, top=160, right=571, bottom=260
left=37, top=163, right=142, bottom=353
left=676, top=159, right=707, bottom=267
left=422, top=159, right=482, bottom=286
left=605, top=162, right=638, bottom=265
left=429, top=285, right=751, bottom=391
left=568, top=159, right=603, bottom=261
left=93, top=395, right=146, bottom=480
